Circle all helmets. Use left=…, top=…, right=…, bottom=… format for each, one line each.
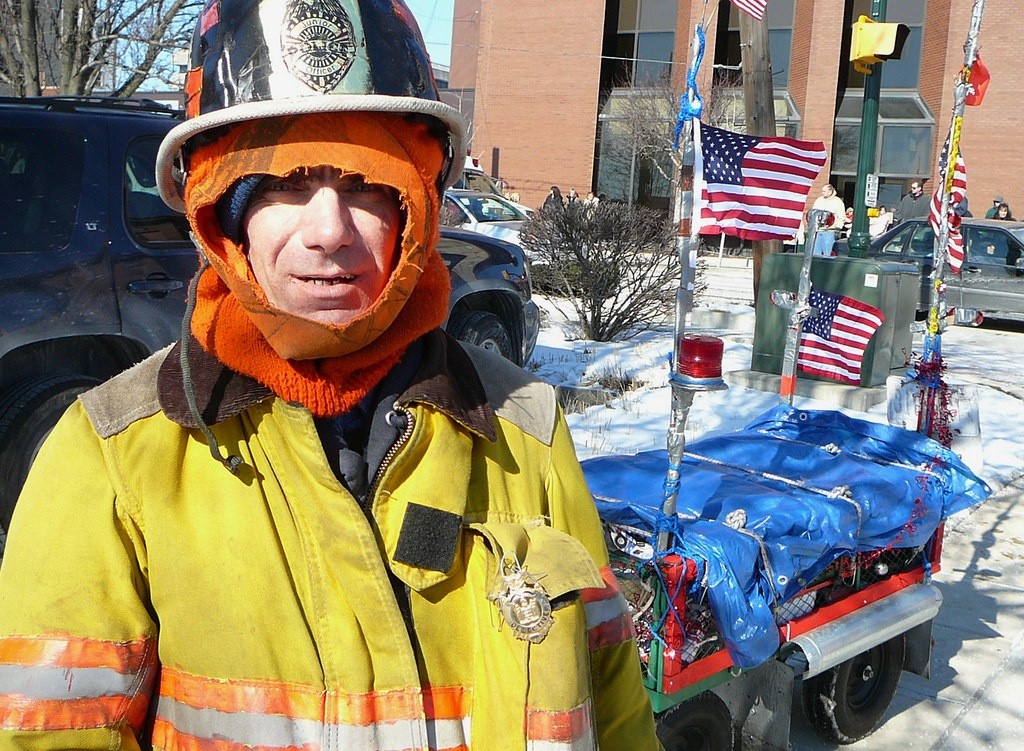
left=154, top=0, right=464, bottom=217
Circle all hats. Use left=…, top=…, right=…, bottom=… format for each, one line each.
left=211, top=172, right=265, bottom=239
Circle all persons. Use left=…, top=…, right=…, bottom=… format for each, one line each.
left=0, top=1, right=666, bottom=751
left=542, top=177, right=1015, bottom=257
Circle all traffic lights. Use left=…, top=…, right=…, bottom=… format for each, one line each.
left=845, top=13, right=910, bottom=75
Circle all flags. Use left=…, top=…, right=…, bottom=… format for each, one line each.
left=731, top=0, right=768, bottom=20
left=930, top=132, right=968, bottom=273
left=797, top=291, right=886, bottom=386
left=961, top=51, right=991, bottom=108
left=691, top=116, right=829, bottom=241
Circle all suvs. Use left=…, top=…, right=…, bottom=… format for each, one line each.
left=0, top=90, right=545, bottom=538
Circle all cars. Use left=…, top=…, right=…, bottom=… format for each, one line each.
left=437, top=150, right=591, bottom=292
left=830, top=217, right=1024, bottom=328
